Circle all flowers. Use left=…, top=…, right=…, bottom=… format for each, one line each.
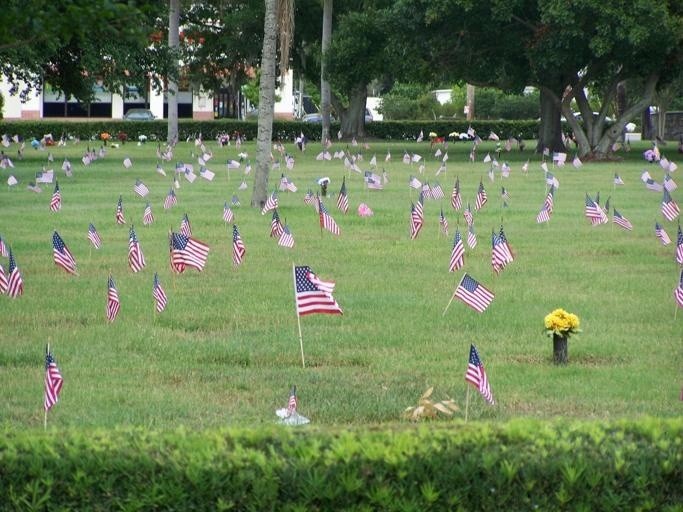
left=429, top=132, right=437, bottom=137
left=449, top=132, right=459, bottom=136
left=117, top=132, right=127, bottom=141
left=541, top=309, right=582, bottom=341
left=101, top=133, right=110, bottom=140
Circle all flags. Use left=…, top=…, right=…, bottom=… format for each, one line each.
left=1, top=121, right=683, bottom=429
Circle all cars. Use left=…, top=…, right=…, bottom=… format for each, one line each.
left=123, top=109, right=155, bottom=123
left=363, top=108, right=373, bottom=123
left=302, top=113, right=333, bottom=123
left=559, top=112, right=612, bottom=120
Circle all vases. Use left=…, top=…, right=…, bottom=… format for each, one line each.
left=122, top=140, right=124, bottom=145
left=104, top=140, right=106, bottom=145
left=432, top=137, right=435, bottom=142
left=553, top=337, right=567, bottom=365
left=453, top=136, right=455, bottom=144
